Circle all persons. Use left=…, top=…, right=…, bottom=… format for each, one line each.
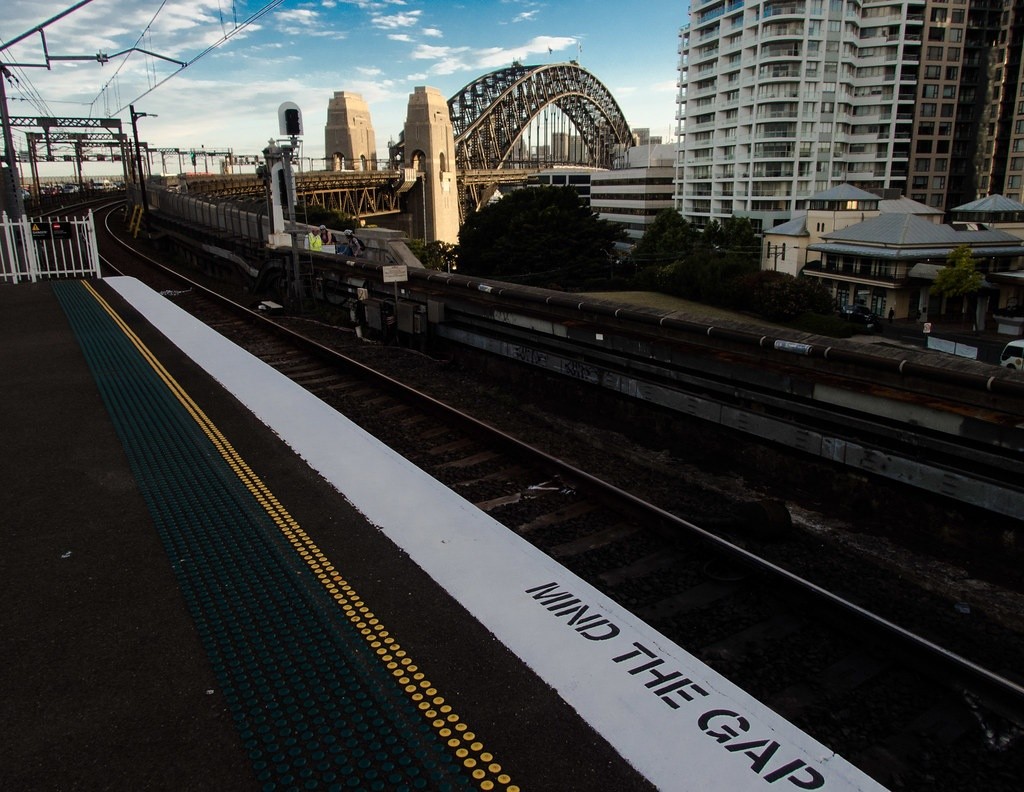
left=305, top=225, right=365, bottom=257
left=888, top=307, right=894, bottom=323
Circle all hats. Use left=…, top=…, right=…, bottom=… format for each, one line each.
left=344, top=229, right=353, bottom=235
left=320, top=225, right=326, bottom=229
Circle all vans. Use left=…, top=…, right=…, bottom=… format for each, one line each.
left=92, top=179, right=115, bottom=191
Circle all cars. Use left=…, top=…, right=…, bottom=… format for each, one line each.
left=62, top=184, right=79, bottom=194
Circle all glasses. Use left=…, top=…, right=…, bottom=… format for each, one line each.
left=321, top=229, right=324, bottom=231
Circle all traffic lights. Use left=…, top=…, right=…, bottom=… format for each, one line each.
left=190, top=152, right=196, bottom=165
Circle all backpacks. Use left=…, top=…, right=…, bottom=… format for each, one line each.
left=349, top=237, right=365, bottom=251
left=321, top=232, right=336, bottom=242
